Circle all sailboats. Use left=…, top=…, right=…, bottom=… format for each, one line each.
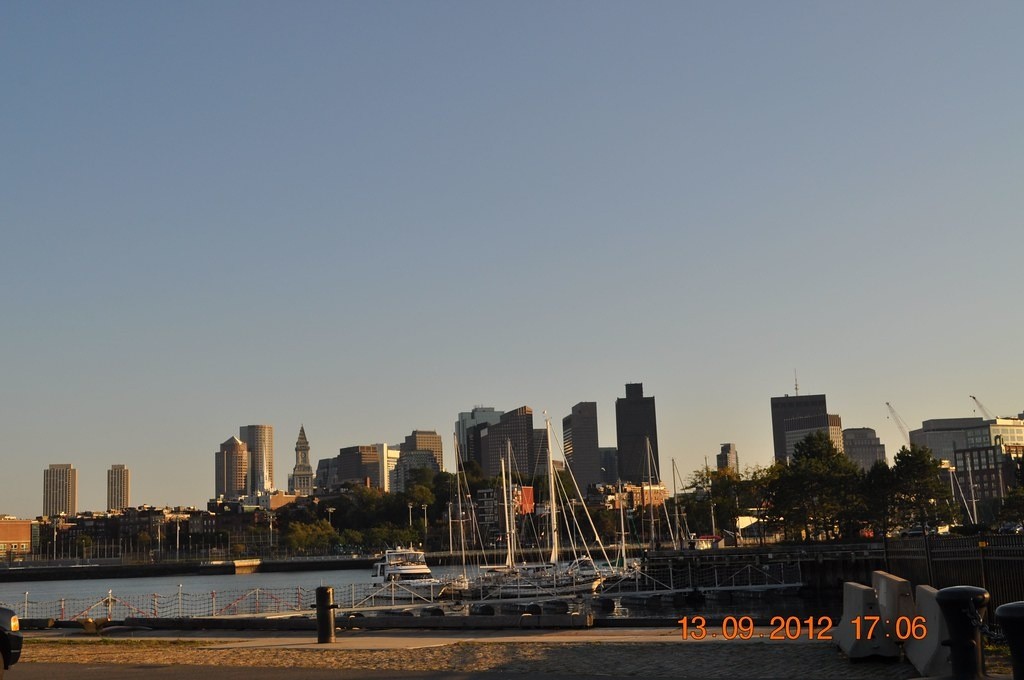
left=438, top=410, right=696, bottom=595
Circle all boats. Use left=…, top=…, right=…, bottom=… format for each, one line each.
left=364, top=543, right=452, bottom=609
left=197, top=559, right=264, bottom=574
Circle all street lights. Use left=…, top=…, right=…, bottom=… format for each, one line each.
left=421, top=504, right=428, bottom=552
left=408, top=503, right=413, bottom=549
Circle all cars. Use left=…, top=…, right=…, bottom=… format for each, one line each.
left=0, top=607, right=24, bottom=680
left=998, top=524, right=1024, bottom=535
left=901, top=527, right=935, bottom=537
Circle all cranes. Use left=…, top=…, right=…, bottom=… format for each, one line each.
left=969, top=394, right=996, bottom=419
left=886, top=402, right=912, bottom=447
